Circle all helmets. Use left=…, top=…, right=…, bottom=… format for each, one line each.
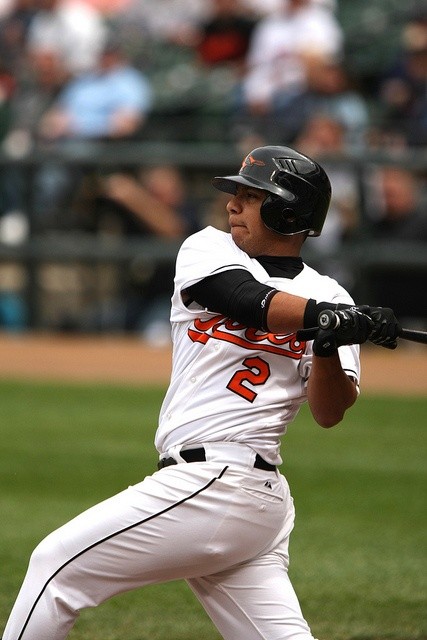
left=213, top=145, right=331, bottom=237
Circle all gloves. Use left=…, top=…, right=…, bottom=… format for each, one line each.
left=302, top=294, right=401, bottom=350
left=311, top=304, right=375, bottom=358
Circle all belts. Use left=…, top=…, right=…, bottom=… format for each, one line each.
left=156, top=447, right=276, bottom=471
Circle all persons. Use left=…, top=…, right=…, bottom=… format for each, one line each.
left=2, top=144, right=397, bottom=640
left=0, top=2, right=426, bottom=242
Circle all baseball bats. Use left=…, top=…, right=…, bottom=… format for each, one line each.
left=319, top=311, right=427, bottom=353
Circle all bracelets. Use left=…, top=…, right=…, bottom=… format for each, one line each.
left=303, top=296, right=336, bottom=331
